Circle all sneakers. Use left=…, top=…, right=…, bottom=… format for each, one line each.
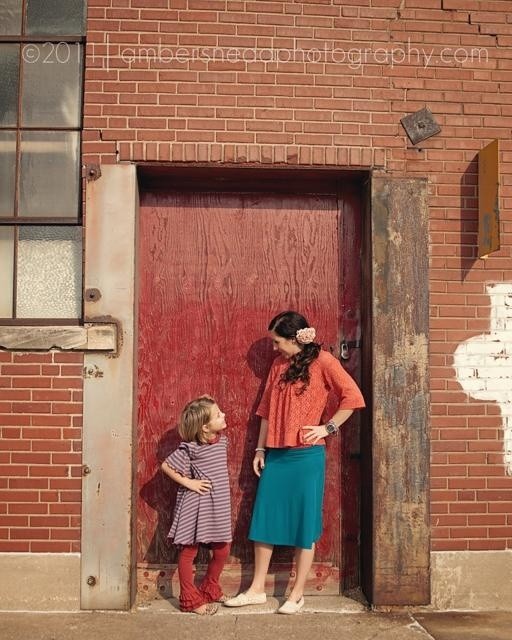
left=277, top=595, right=305, bottom=615
left=224, top=590, right=267, bottom=607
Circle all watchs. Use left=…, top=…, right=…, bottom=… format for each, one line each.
left=325, top=419, right=338, bottom=435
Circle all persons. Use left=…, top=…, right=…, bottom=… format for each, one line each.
left=222, top=310, right=366, bottom=615
left=159, top=395, right=234, bottom=614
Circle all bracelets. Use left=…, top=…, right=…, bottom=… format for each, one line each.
left=254, top=447, right=266, bottom=452
